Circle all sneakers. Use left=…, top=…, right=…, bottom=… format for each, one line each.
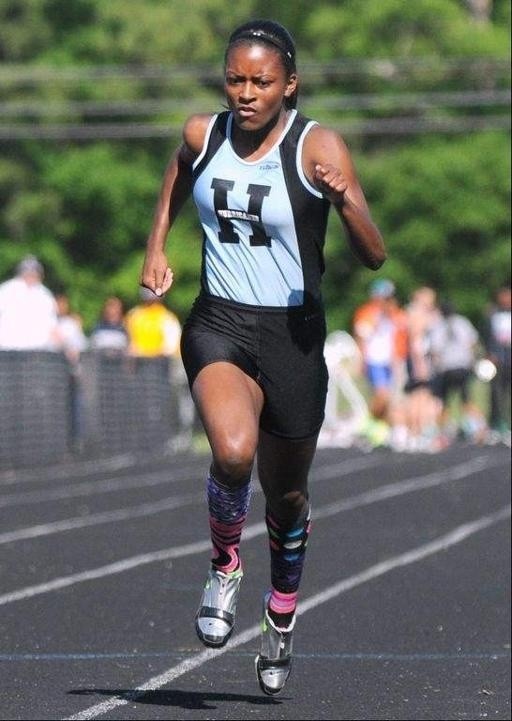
left=194, top=566, right=244, bottom=649
left=254, top=593, right=295, bottom=697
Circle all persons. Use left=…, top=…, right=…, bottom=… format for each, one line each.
left=137, top=19, right=386, bottom=697
left=0, top=252, right=511, bottom=461
